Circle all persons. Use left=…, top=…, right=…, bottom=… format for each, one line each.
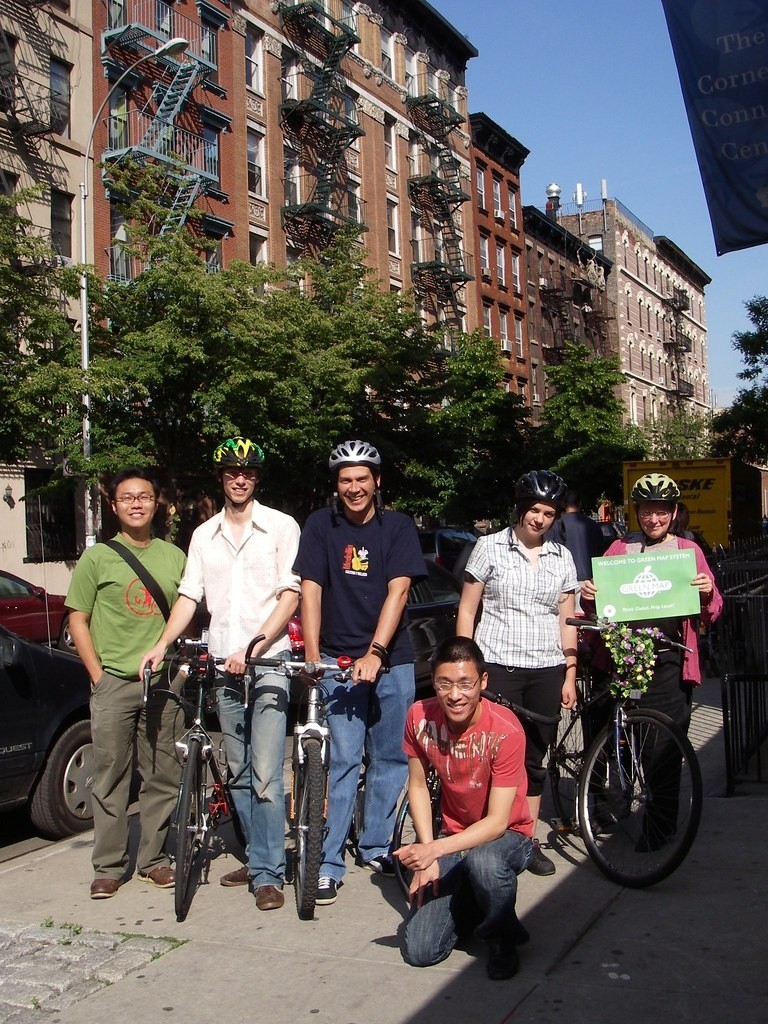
left=580, top=473, right=724, bottom=853
left=668, top=503, right=712, bottom=561
left=392, top=637, right=534, bottom=980
left=292, top=439, right=429, bottom=905
left=64, top=468, right=196, bottom=898
left=544, top=489, right=604, bottom=615
left=139, top=437, right=302, bottom=911
left=455, top=470, right=581, bottom=876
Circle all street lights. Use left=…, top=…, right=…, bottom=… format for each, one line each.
left=78, top=34, right=190, bottom=546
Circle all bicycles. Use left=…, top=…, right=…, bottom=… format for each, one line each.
left=141, top=638, right=245, bottom=918
left=246, top=635, right=391, bottom=921
left=391, top=689, right=564, bottom=903
left=544, top=616, right=706, bottom=890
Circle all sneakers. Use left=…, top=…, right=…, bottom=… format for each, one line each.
left=634, top=833, right=666, bottom=852
left=315, top=877, right=344, bottom=905
left=370, top=854, right=396, bottom=877
left=484, top=945, right=520, bottom=981
left=527, top=838, right=556, bottom=876
left=589, top=815, right=611, bottom=835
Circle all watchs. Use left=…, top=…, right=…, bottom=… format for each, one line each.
left=177, top=664, right=192, bottom=676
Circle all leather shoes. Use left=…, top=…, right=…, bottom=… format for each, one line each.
left=255, top=885, right=284, bottom=910
left=137, top=867, right=176, bottom=888
left=220, top=866, right=250, bottom=886
left=91, top=876, right=132, bottom=899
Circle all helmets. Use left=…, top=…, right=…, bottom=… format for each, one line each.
left=514, top=470, right=569, bottom=513
left=212, top=436, right=266, bottom=468
left=329, top=440, right=381, bottom=473
left=630, top=472, right=681, bottom=503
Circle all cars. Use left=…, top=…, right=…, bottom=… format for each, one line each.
left=0, top=623, right=94, bottom=841
left=405, top=526, right=484, bottom=699
left=0, top=568, right=80, bottom=656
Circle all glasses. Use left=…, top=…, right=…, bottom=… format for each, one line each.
left=433, top=674, right=482, bottom=690
left=637, top=508, right=672, bottom=518
left=223, top=470, right=256, bottom=478
left=114, top=495, right=156, bottom=503
